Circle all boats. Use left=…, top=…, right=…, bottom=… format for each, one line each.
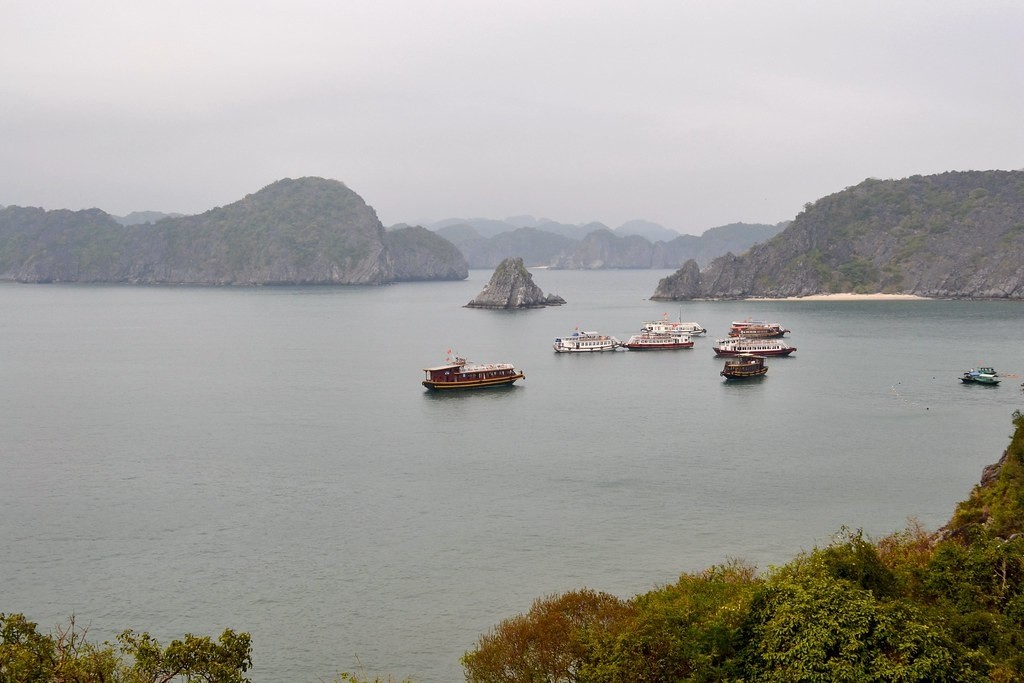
left=720, top=354, right=769, bottom=380
left=625, top=332, right=694, bottom=350
left=552, top=329, right=621, bottom=352
left=641, top=304, right=707, bottom=337
left=422, top=349, right=525, bottom=391
left=712, top=336, right=797, bottom=358
left=957, top=365, right=1002, bottom=387
left=728, top=321, right=791, bottom=339
